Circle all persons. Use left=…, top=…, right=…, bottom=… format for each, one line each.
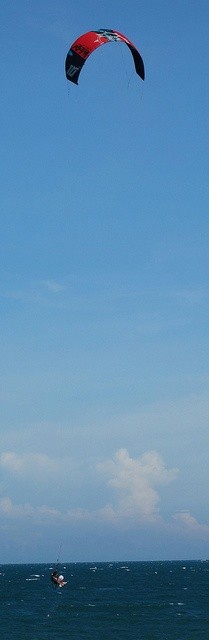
left=51, top=572, right=67, bottom=588
